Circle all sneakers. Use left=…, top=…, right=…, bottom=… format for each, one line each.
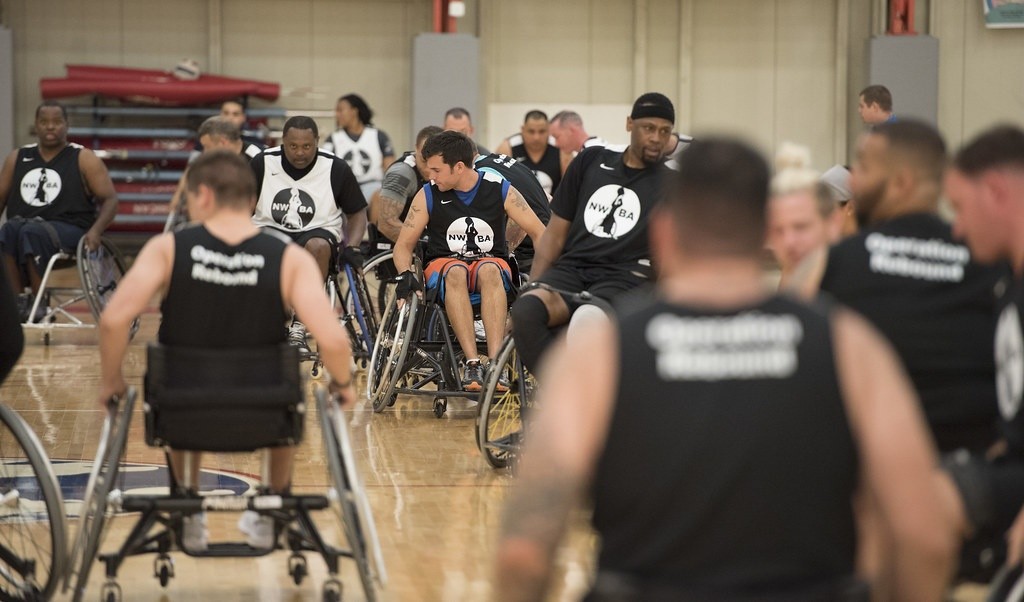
left=472, top=320, right=486, bottom=340
left=182, top=509, right=208, bottom=553
left=237, top=507, right=275, bottom=550
left=462, top=360, right=486, bottom=391
left=25, top=292, right=47, bottom=320
left=483, top=357, right=509, bottom=392
left=18, top=292, right=31, bottom=322
left=287, top=313, right=307, bottom=348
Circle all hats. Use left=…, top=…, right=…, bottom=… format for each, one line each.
left=632, top=92, right=674, bottom=125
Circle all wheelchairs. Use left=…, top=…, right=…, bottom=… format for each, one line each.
left=0, top=399, right=67, bottom=602
left=279, top=222, right=541, bottom=478
left=62, top=342, right=388, bottom=601
left=22, top=233, right=140, bottom=347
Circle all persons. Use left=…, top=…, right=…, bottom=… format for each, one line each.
left=0, top=260, right=26, bottom=382
left=98, top=148, right=358, bottom=554
left=153, top=93, right=1023, bottom=602
left=0, top=100, right=118, bottom=325
